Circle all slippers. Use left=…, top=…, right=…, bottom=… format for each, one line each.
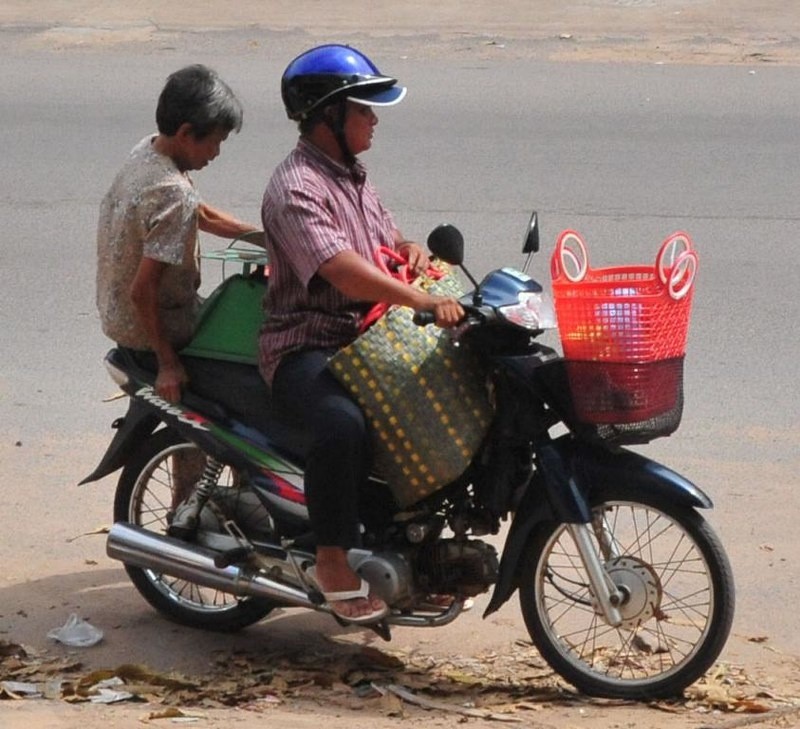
left=304, top=565, right=388, bottom=625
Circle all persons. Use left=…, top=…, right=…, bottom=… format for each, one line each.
left=94, top=63, right=266, bottom=514
left=258, top=44, right=465, bottom=626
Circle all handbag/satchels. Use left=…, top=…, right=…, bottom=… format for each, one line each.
left=326, top=246, right=489, bottom=506
left=551, top=230, right=698, bottom=426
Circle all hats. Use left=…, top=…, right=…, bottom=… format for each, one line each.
left=346, top=83, right=407, bottom=106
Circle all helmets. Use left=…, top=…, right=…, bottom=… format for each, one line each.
left=281, top=43, right=397, bottom=120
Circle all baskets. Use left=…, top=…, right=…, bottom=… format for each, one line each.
left=546, top=357, right=685, bottom=444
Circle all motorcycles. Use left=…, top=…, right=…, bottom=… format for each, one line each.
left=75, top=210, right=737, bottom=702
